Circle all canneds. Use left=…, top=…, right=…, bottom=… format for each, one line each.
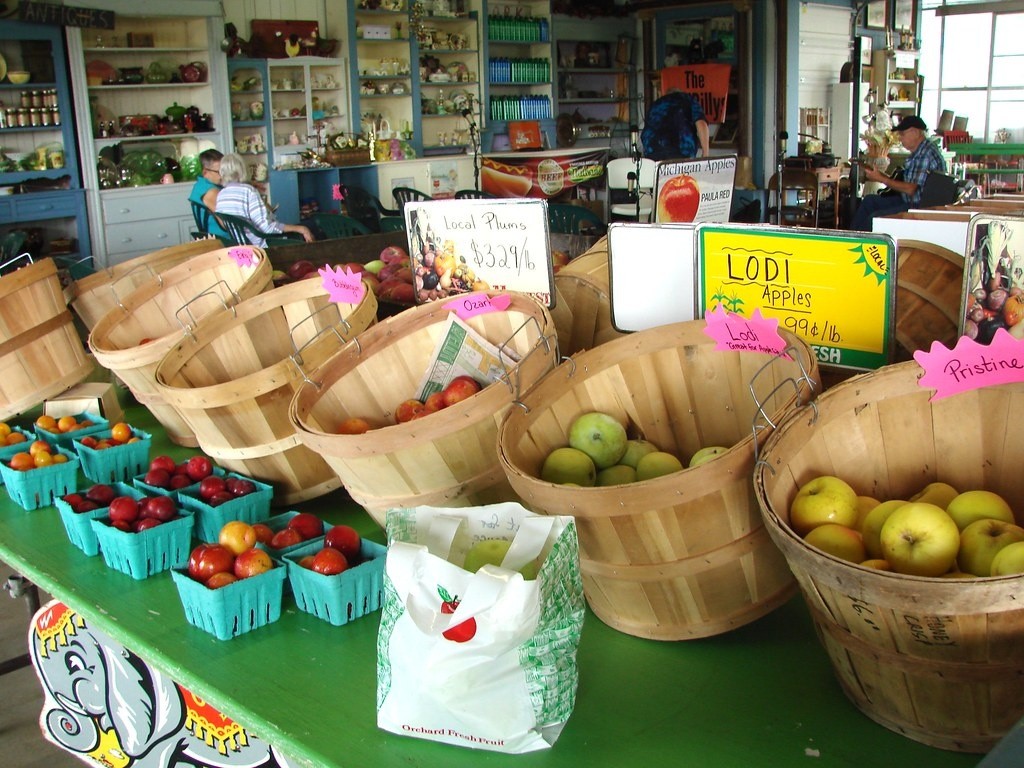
left=0, top=88, right=60, bottom=129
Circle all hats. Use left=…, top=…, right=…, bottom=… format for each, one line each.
left=891, top=116, right=926, bottom=131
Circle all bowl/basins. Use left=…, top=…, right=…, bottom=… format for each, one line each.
left=7, top=72, right=30, bottom=84
left=429, top=74, right=450, bottom=82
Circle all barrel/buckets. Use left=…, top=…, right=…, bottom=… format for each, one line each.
left=88, top=244, right=276, bottom=449
left=155, top=277, right=379, bottom=501
left=289, top=289, right=559, bottom=532
left=498, top=318, right=825, bottom=642
left=754, top=360, right=1024, bottom=755
left=0, top=253, right=94, bottom=421
left=65, top=238, right=225, bottom=333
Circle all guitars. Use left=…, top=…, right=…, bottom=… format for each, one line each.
left=849, top=156, right=905, bottom=196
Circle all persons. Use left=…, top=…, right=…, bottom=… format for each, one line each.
left=189, top=149, right=231, bottom=240
left=640, top=88, right=709, bottom=163
left=215, top=153, right=318, bottom=248
left=850, top=115, right=946, bottom=231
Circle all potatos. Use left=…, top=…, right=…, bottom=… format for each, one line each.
left=418, top=289, right=447, bottom=302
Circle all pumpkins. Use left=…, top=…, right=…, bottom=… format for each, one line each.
left=423, top=252, right=490, bottom=292
left=976, top=293, right=1024, bottom=345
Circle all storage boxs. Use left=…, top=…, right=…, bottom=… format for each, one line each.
left=170, top=554, right=287, bottom=641
left=0, top=443, right=80, bottom=512
left=72, top=424, right=152, bottom=484
left=90, top=509, right=195, bottom=580
left=281, top=538, right=388, bottom=626
left=251, top=511, right=335, bottom=561
left=871, top=192, right=1024, bottom=257
left=132, top=460, right=226, bottom=502
left=53, top=481, right=149, bottom=557
left=33, top=410, right=110, bottom=457
left=0, top=425, right=37, bottom=484
left=178, top=472, right=274, bottom=544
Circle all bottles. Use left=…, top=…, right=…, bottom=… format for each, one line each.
left=489, top=56, right=550, bottom=84
left=100, top=122, right=107, bottom=138
left=489, top=14, right=549, bottom=42
left=908, top=30, right=914, bottom=50
left=108, top=121, right=115, bottom=136
left=900, top=25, right=906, bottom=49
left=490, top=95, right=551, bottom=120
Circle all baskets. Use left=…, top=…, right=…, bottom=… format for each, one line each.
left=377, top=119, right=394, bottom=140
left=0, top=410, right=386, bottom=639
left=326, top=133, right=371, bottom=166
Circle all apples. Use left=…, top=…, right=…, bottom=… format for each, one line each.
left=542, top=413, right=733, bottom=488
left=791, top=475, right=1024, bottom=578
left=971, top=287, right=1021, bottom=321
left=416, top=267, right=426, bottom=275
left=658, top=175, right=700, bottom=223
left=272, top=245, right=415, bottom=305
left=338, top=376, right=479, bottom=435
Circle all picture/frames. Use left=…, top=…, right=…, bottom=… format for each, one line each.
left=860, top=35, right=873, bottom=66
left=893, top=0, right=915, bottom=34
left=864, top=0, right=889, bottom=31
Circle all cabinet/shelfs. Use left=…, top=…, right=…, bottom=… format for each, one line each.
left=0, top=19, right=97, bottom=276
left=869, top=49, right=921, bottom=174
left=268, top=162, right=381, bottom=226
left=326, top=0, right=636, bottom=162
left=266, top=55, right=349, bottom=168
left=62, top=0, right=229, bottom=273
left=227, top=58, right=272, bottom=184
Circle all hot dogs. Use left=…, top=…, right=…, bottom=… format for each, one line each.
left=481, top=158, right=533, bottom=198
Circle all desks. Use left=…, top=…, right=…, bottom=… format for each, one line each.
left=782, top=166, right=842, bottom=230
left=0, top=388, right=985, bottom=768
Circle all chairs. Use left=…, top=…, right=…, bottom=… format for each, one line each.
left=942, top=130, right=988, bottom=194
left=188, top=184, right=606, bottom=248
left=604, top=157, right=656, bottom=228
left=766, top=169, right=820, bottom=228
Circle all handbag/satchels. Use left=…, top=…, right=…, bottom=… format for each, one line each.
left=877, top=166, right=905, bottom=196
left=790, top=153, right=833, bottom=166
left=376, top=502, right=586, bottom=755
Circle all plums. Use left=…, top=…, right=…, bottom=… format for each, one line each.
left=0, top=415, right=256, bottom=533
left=188, top=512, right=359, bottom=591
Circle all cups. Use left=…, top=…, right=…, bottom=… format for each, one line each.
left=160, top=174, right=173, bottom=184
